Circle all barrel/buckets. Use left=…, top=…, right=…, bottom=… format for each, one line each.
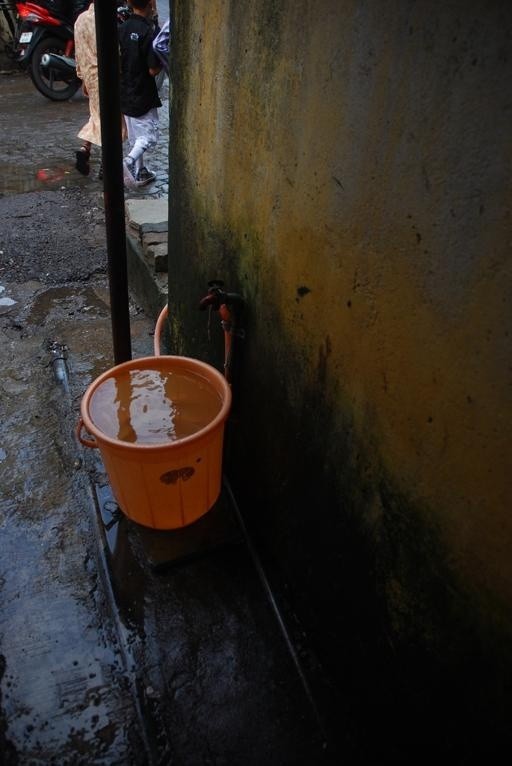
left=76, top=355, right=231, bottom=530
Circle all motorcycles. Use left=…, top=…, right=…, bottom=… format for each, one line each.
left=0, top=0, right=167, bottom=102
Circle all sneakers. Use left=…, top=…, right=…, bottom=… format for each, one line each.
left=136, top=167, right=156, bottom=186
left=76, top=145, right=90, bottom=175
left=123, top=156, right=136, bottom=190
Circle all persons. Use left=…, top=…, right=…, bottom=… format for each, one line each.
left=150, top=12, right=171, bottom=81
left=68, top=0, right=126, bottom=182
left=115, top=1, right=163, bottom=188
